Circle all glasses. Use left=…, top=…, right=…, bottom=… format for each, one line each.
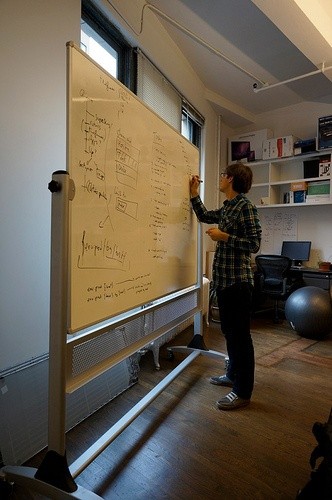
left=220, top=172, right=227, bottom=177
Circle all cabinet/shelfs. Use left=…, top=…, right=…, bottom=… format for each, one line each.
left=244, top=149, right=332, bottom=209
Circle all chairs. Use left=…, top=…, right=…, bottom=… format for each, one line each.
left=254, top=254, right=292, bottom=324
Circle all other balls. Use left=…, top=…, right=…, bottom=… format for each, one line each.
left=285, top=286, right=332, bottom=341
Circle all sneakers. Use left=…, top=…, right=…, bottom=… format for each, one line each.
left=210, top=375, right=234, bottom=387
left=217, top=391, right=250, bottom=410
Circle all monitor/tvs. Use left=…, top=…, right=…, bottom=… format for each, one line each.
left=231, top=141, right=256, bottom=164
left=281, top=241, right=311, bottom=269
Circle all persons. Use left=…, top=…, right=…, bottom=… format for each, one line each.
left=191, top=163, right=262, bottom=409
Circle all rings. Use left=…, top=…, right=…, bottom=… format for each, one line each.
left=209, top=232, right=211, bottom=236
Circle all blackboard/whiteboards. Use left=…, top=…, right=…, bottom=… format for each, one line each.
left=66, top=41, right=200, bottom=334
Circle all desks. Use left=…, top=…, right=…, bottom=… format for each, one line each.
left=292, top=267, right=332, bottom=294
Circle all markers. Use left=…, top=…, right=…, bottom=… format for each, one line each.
left=191, top=175, right=203, bottom=183
left=142, top=302, right=153, bottom=309
